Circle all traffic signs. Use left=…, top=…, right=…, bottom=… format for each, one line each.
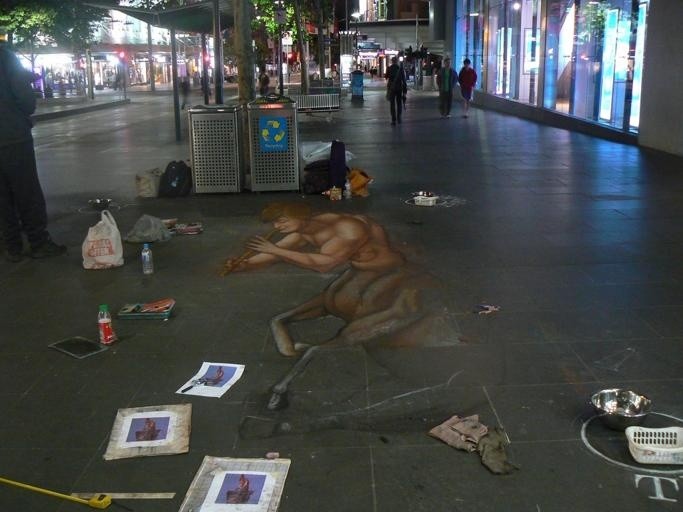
left=272, top=7, right=287, bottom=25
left=356, top=33, right=381, bottom=50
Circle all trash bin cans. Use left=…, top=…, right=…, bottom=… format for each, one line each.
left=247, top=93, right=298, bottom=192
left=187, top=102, right=243, bottom=194
left=353, top=71, right=363, bottom=96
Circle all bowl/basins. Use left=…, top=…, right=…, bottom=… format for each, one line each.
left=591, top=388, right=653, bottom=431
left=86, top=197, right=112, bottom=210
left=408, top=191, right=440, bottom=207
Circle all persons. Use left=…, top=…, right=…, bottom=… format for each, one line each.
left=360, top=64, right=378, bottom=78
left=436, top=58, right=458, bottom=120
left=457, top=58, right=477, bottom=118
left=0, top=47, right=67, bottom=263
left=199, top=72, right=213, bottom=101
left=216, top=200, right=412, bottom=274
left=383, top=56, right=407, bottom=128
left=257, top=66, right=269, bottom=95
left=178, top=75, right=194, bottom=110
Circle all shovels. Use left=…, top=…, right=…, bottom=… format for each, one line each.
left=182, top=379, right=206, bottom=393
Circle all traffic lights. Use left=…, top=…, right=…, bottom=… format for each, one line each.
left=420, top=45, right=427, bottom=60
left=412, top=50, right=420, bottom=58
left=405, top=45, right=412, bottom=57
left=118, top=49, right=125, bottom=63
left=205, top=54, right=210, bottom=66
left=287, top=51, right=293, bottom=65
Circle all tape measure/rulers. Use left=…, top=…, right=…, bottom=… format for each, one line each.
left=70, top=493, right=176, bottom=500
left=0, top=478, right=111, bottom=510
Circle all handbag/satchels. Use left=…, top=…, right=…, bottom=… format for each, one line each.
left=386, top=88, right=393, bottom=101
left=160, top=160, right=192, bottom=198
left=135, top=167, right=161, bottom=199
left=348, top=167, right=372, bottom=197
left=303, top=160, right=330, bottom=194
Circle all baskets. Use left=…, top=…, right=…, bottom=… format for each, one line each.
left=414, top=195, right=438, bottom=207
left=626, top=425, right=683, bottom=466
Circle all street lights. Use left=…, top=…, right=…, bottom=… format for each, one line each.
left=350, top=8, right=361, bottom=71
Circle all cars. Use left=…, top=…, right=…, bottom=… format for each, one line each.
left=308, top=63, right=336, bottom=81
left=192, top=63, right=300, bottom=82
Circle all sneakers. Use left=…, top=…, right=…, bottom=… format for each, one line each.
left=31, top=243, right=50, bottom=257
left=6, top=246, right=22, bottom=263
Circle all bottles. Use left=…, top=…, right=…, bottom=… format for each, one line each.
left=94, top=302, right=115, bottom=345
left=344, top=179, right=353, bottom=201
left=140, top=243, right=154, bottom=275
